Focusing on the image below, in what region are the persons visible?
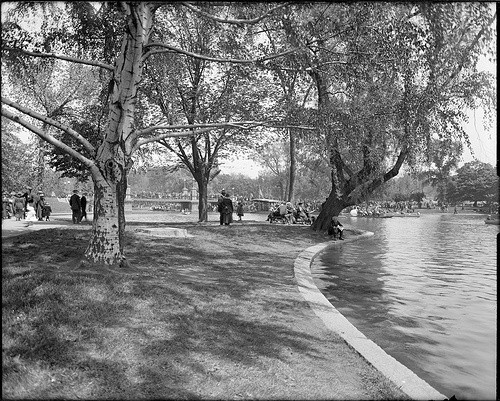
[80,192,87,222]
[354,204,415,216]
[2,187,52,221]
[328,216,345,241]
[266,201,310,224]
[236,202,244,220]
[69,189,82,224]
[217,190,233,225]
